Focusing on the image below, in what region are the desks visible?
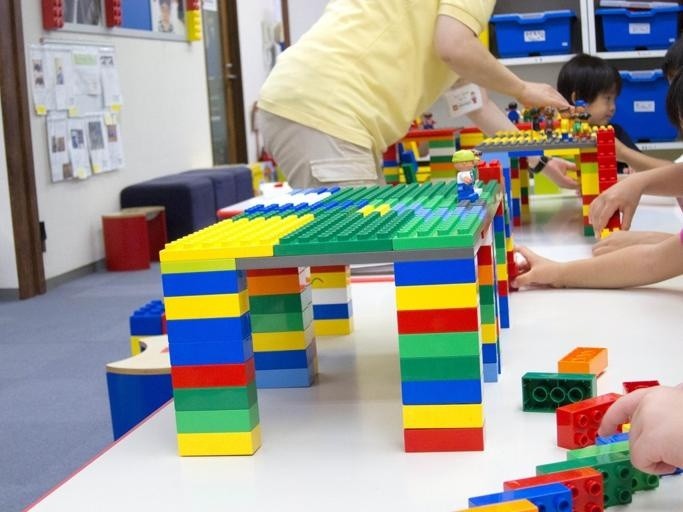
[21,177,682,511]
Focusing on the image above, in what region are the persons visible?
[512,41,683,287]
[157,0,175,33]
[616,35,683,171]
[588,67,683,257]
[557,54,641,174]
[597,382,683,474]
[256,0,581,191]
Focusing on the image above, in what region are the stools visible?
[100,166,253,272]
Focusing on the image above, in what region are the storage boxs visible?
[489,0,682,143]
